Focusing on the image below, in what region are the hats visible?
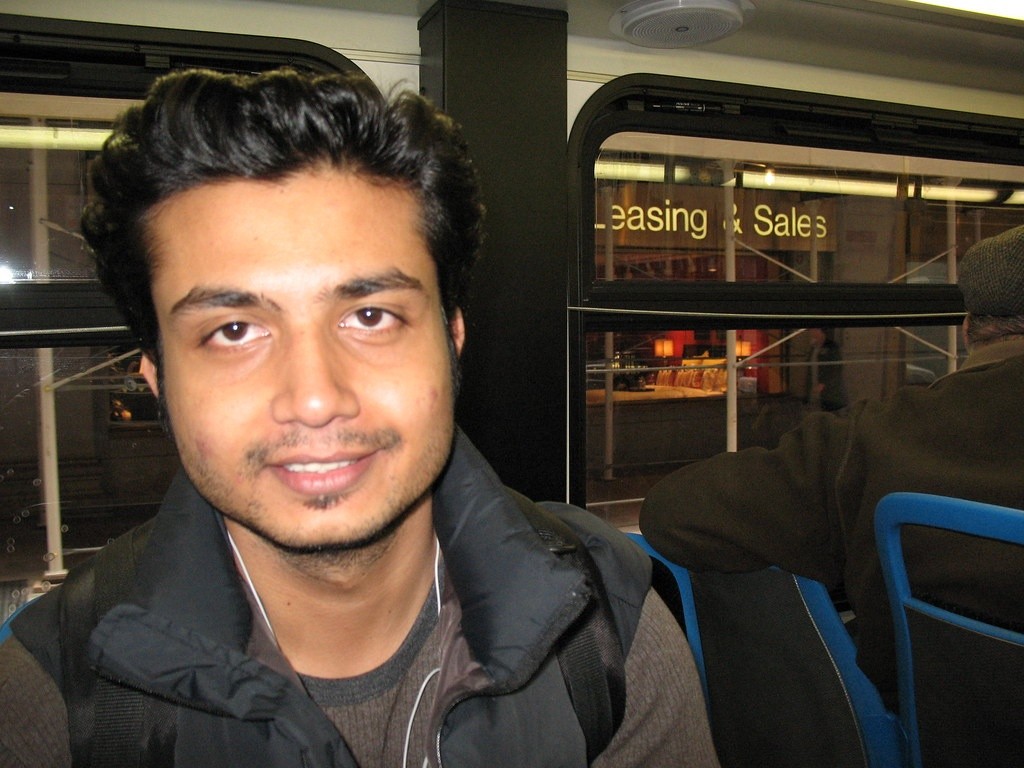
[957,225,1024,316]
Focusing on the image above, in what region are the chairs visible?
[626,491,1023,768]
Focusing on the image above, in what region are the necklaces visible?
[221,528,443,768]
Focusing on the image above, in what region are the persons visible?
[804,328,848,413]
[637,225,1024,768]
[0,71,719,768]
